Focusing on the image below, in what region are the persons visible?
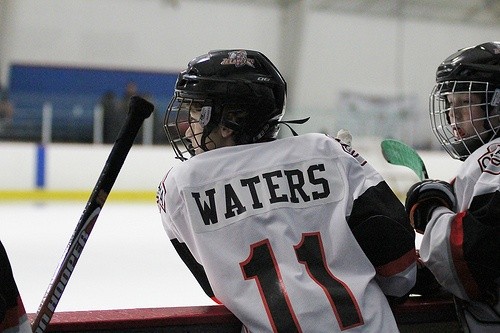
[404,41,500,333]
[102,78,144,144]
[153,47,419,333]
[0,242,34,333]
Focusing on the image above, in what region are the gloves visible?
[405,179,458,235]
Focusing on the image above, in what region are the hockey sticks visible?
[380,139,429,181]
[30,94,155,333]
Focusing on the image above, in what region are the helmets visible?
[162,49,287,162]
[429,42,500,161]
